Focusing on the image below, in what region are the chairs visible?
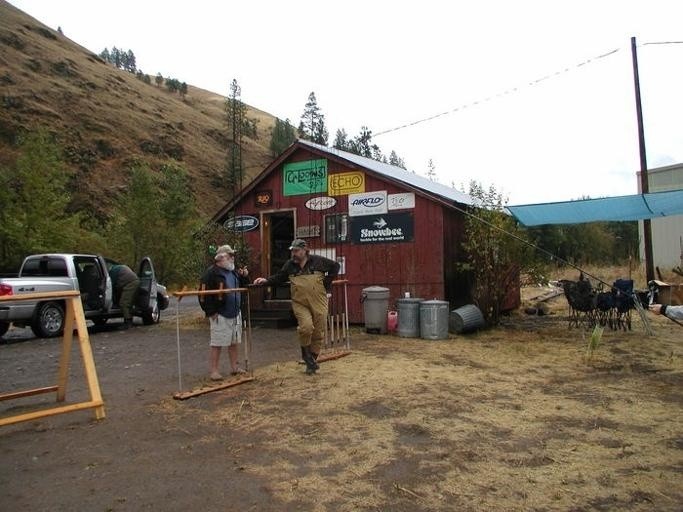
[560,278,634,332]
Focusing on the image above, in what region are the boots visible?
[301,345,318,369]
[306,352,318,375]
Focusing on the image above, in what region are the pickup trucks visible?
[0,253,169,339]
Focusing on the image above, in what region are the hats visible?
[216,245,235,254]
[289,239,305,248]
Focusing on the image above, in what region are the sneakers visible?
[210,372,221,380]
[230,368,245,373]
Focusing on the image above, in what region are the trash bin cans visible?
[450,304,483,335]
[362,285,390,334]
[396,298,450,339]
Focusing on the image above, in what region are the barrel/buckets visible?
[387,309,398,331]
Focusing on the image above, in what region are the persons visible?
[106,264,140,331]
[197,244,249,380]
[252,238,340,375]
[648,303,683,320]
